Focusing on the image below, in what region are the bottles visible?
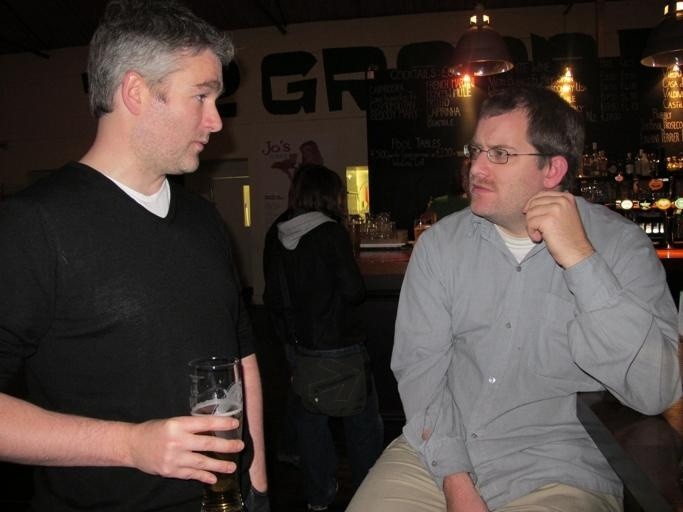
[579,141,683,243]
[351,211,395,241]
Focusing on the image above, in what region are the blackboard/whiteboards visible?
[366,53,683,242]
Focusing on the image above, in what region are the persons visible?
[659,291,683,438]
[0,6,273,510]
[262,163,385,512]
[343,82,683,512]
[428,159,469,222]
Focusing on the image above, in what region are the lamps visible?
[446,1,513,78]
[639,1,682,67]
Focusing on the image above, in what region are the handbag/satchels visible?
[288,341,370,419]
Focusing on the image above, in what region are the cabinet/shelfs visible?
[565,169,682,250]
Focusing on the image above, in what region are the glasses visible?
[463,142,553,166]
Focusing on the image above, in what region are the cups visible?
[189,355,246,511]
[414,218,433,241]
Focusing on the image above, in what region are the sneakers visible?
[308,476,339,511]
